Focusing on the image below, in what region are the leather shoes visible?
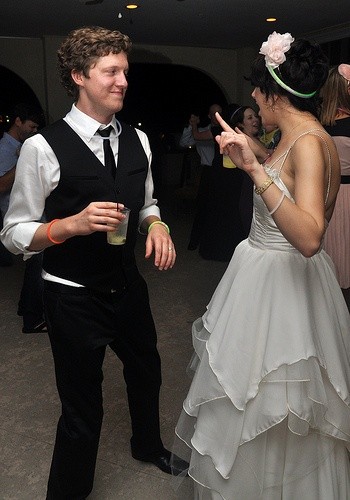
[131,441,190,477]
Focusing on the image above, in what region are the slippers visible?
[22,320,48,334]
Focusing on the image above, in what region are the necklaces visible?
[72,115,123,140]
[273,116,318,153]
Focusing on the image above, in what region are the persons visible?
[217,106,258,259]
[0,112,50,334]
[318,64,350,311]
[1,27,192,499]
[174,32,350,500]
[191,102,221,256]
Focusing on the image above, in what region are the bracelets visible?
[269,190,285,218]
[148,221,170,235]
[48,218,65,243]
[255,177,273,196]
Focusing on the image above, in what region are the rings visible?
[169,247,174,251]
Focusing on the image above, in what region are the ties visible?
[95,125,118,180]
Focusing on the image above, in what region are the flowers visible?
[259,31,294,69]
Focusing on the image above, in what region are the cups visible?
[107,207,132,246]
